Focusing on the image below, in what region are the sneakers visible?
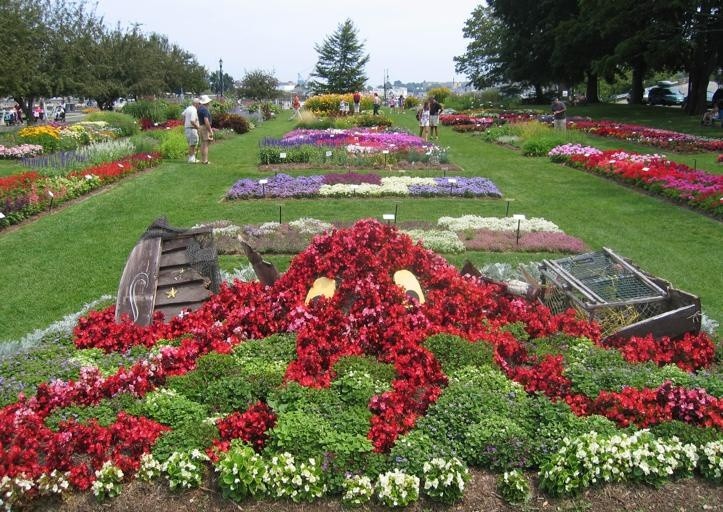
[435,136,438,139]
[202,161,211,165]
[188,160,200,163]
[430,136,433,139]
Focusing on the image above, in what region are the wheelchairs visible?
[701,105,723,128]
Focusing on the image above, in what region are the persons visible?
[399,95,406,114]
[429,98,443,139]
[372,93,380,116]
[196,94,214,164]
[551,96,567,133]
[4,101,66,126]
[288,94,302,120]
[389,96,397,114]
[703,103,719,127]
[180,98,200,163]
[339,98,348,116]
[354,90,361,115]
[418,101,432,142]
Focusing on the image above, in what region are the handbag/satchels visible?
[416,109,423,121]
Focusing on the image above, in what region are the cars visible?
[682,91,715,109]
[626,89,649,105]
[648,87,686,106]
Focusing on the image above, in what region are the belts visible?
[186,127,197,129]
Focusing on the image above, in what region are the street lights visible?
[219,59,223,96]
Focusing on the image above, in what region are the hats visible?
[199,95,212,104]
[551,96,557,101]
